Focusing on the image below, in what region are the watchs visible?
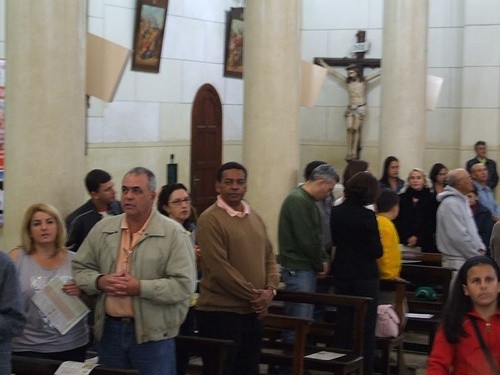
[268,287,276,296]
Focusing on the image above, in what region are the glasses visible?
[169,197,191,206]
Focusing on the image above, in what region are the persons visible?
[0,140,500,375]
[319,58,382,162]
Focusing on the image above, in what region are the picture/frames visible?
[223,6,244,79]
[132,0,169,74]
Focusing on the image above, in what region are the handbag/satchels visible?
[375,305,401,337]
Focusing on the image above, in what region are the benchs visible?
[11,264,454,375]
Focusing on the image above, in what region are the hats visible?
[414,286,435,301]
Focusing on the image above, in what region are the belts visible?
[107,315,134,323]
